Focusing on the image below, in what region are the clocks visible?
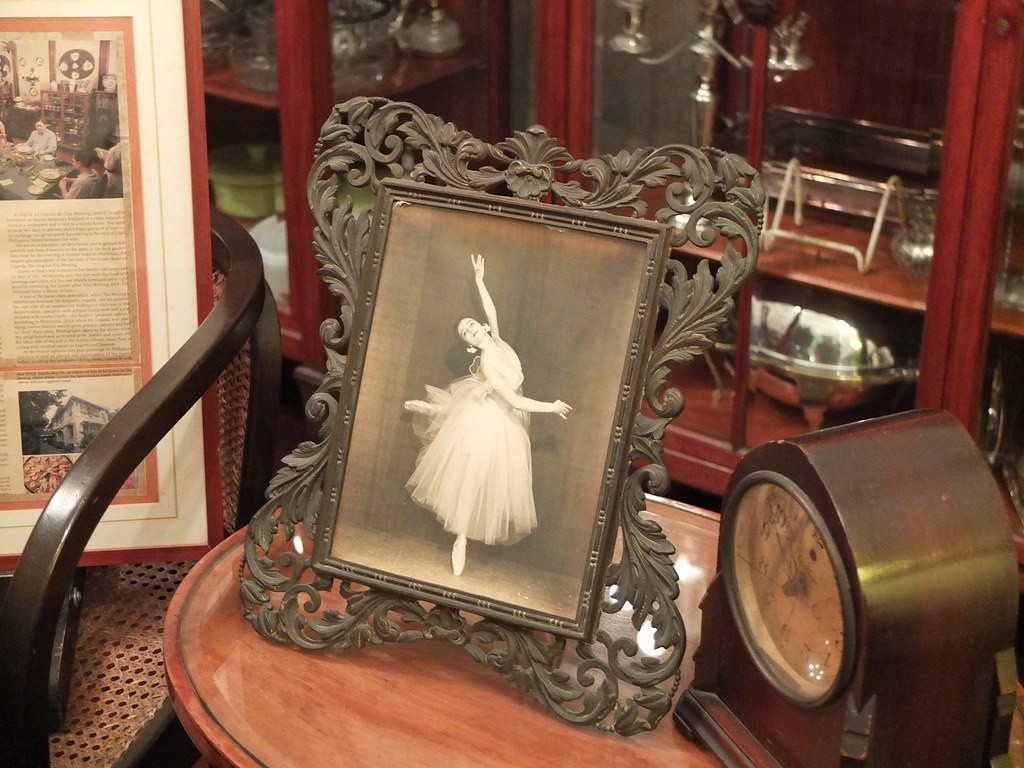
[673,407,1024,768]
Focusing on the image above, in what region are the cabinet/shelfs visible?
[203,0,513,441]
[535,0,1024,594]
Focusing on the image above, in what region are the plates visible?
[15,143,33,155]
[28,185,44,195]
[43,154,54,161]
[39,169,60,182]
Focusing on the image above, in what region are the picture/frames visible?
[237,98,765,736]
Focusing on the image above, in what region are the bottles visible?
[50,78,87,93]
[230,22,278,91]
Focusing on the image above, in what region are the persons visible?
[404,253,575,576]
[16,116,123,199]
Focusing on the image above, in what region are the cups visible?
[1,160,6,165]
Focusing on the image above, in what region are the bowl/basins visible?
[212,141,278,217]
[247,0,398,68]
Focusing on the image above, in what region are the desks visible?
[163,494,721,768]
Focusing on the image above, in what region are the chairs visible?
[0,209,283,768]
